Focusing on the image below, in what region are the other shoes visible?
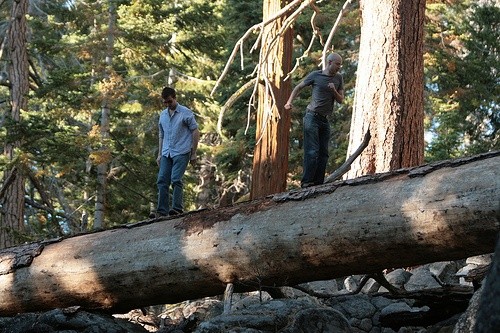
[149,211,167,219]
[169,208,183,215]
[303,182,314,187]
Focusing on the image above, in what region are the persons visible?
[284,53,345,188]
[148,87,200,218]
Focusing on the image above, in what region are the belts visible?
[309,111,329,119]
